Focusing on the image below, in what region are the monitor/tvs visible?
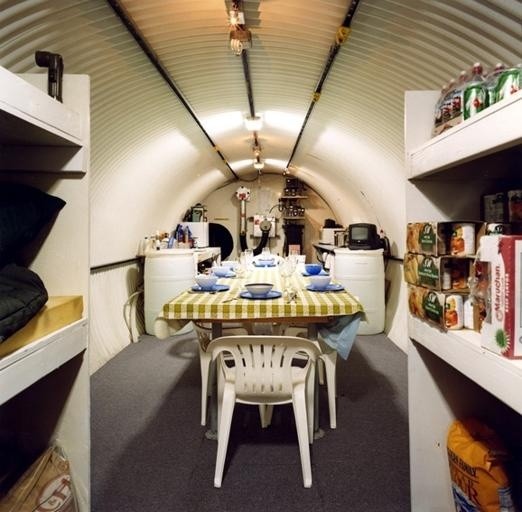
[348,222,377,250]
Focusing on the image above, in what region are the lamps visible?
[245,116,262,131]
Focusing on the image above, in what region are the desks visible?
[312,241,338,253]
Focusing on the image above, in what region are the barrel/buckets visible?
[143,250,194,336]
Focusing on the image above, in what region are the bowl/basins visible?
[245,283,274,298]
[310,276,331,290]
[195,275,218,290]
[305,265,322,275]
[212,266,230,277]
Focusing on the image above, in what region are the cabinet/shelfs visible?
[403,87,521,512]
[1,65,90,512]
[143,247,222,337]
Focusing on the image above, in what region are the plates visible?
[192,285,229,291]
[240,290,282,299]
[255,264,276,267]
[211,273,236,278]
[306,284,344,292]
[300,269,329,276]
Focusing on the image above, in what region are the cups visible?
[262,247,270,271]
[280,256,298,293]
[240,249,253,275]
[298,254,308,266]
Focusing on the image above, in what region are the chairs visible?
[193,320,214,426]
[204,334,322,490]
[317,340,339,430]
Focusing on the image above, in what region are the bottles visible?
[433,62,522,137]
[143,237,149,254]
[162,233,169,248]
[150,236,156,252]
[156,234,161,251]
